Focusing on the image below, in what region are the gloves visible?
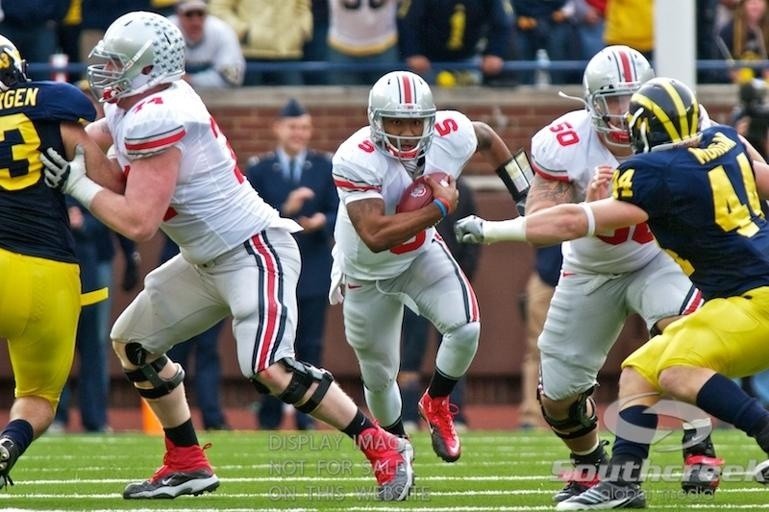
[455,215,525,244]
[39,144,104,210]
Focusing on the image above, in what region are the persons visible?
[1,1,768,512]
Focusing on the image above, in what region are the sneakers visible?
[359,388,460,501]
[681,436,721,493]
[123,438,219,498]
[555,440,645,511]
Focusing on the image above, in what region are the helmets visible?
[368,70,436,160]
[87,11,186,103]
[0,35,31,92]
[584,44,700,154]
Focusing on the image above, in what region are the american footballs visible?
[396,172,449,213]
